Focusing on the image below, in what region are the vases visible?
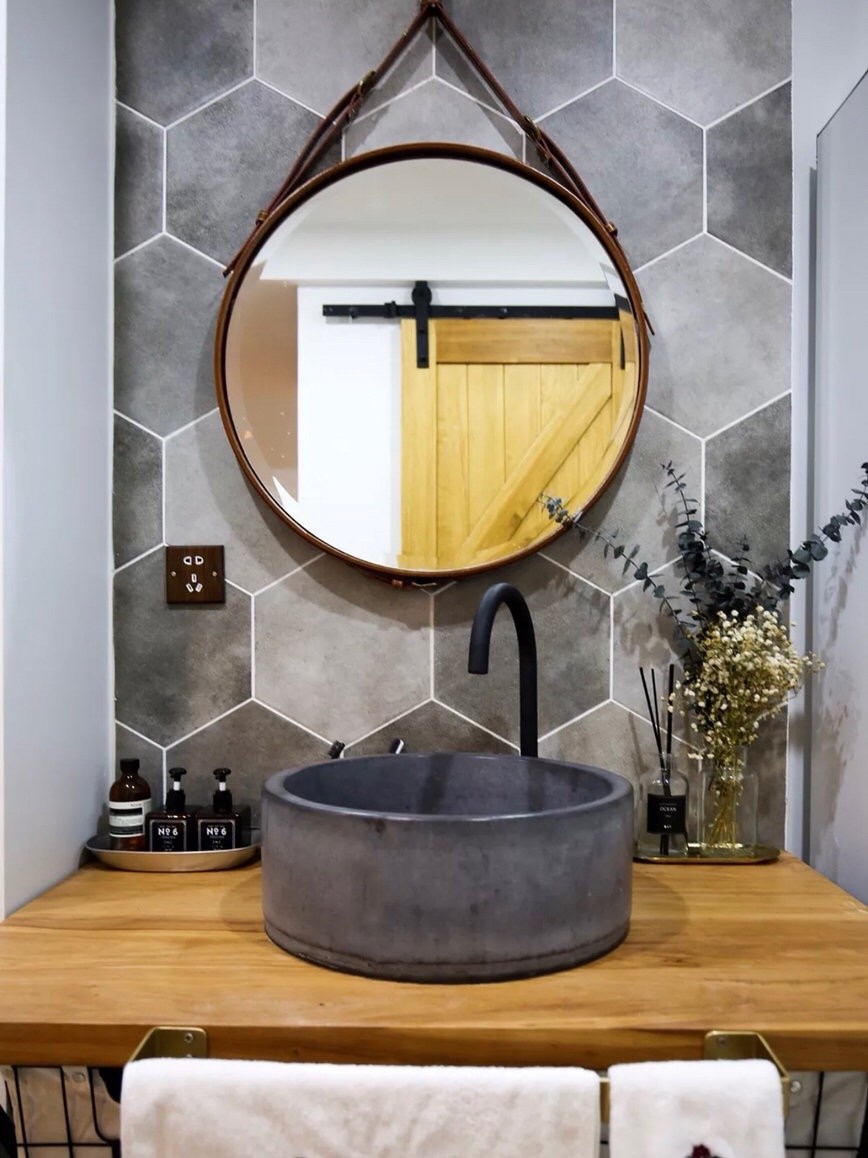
[697,746,758,857]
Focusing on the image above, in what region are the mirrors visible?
[213,0,655,590]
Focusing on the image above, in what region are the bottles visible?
[108,759,152,852]
[637,753,689,857]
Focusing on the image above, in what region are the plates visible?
[85,832,262,872]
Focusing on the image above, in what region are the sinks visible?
[255,751,642,986]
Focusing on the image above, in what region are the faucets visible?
[468,582,540,758]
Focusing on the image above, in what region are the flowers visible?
[656,604,826,849]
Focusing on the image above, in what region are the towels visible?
[118,1054,602,1158]
[602,1056,790,1158]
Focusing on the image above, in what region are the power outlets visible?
[166,545,225,604]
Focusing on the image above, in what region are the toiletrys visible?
[146,767,205,854]
[197,765,253,851]
